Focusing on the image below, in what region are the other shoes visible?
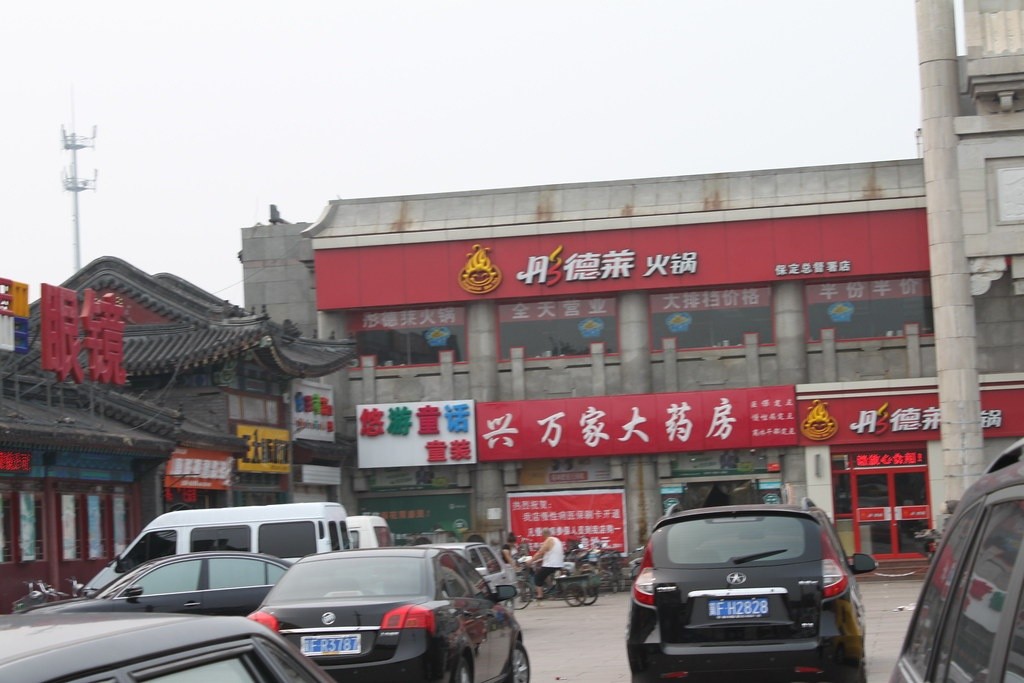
[533,597,546,600]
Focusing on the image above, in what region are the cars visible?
[16,549,293,619]
[245,546,531,683]
[0,610,336,683]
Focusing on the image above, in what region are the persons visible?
[527,528,563,599]
[499,535,517,567]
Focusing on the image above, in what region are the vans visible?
[347,515,394,549]
[83,501,353,598]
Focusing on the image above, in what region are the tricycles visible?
[508,558,597,611]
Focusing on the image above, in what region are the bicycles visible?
[12,576,84,614]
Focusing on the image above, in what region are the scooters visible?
[630,546,645,582]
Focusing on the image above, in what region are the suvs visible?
[893,438,1024,683]
[625,498,878,683]
